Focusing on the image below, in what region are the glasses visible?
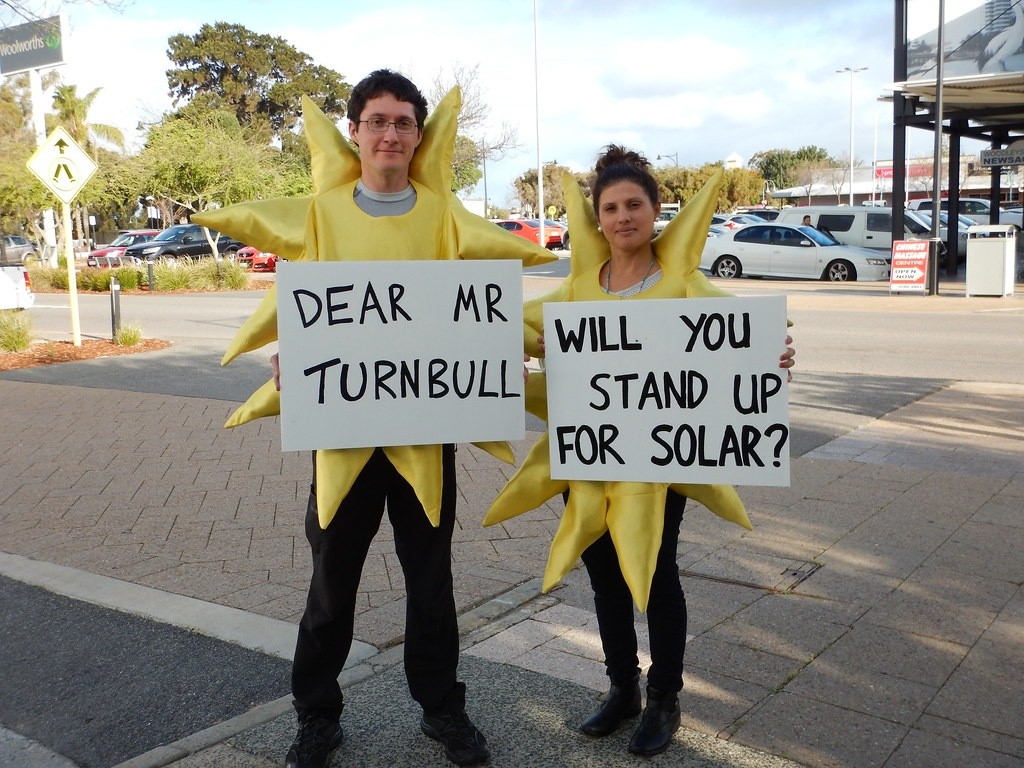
[358,117,420,134]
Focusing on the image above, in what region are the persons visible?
[536,145,796,760]
[976,0,1024,73]
[802,215,815,228]
[271,70,530,768]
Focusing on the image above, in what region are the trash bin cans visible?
[966,225,1015,298]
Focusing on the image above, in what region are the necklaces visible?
[605,257,656,295]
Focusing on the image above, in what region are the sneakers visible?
[420,709,489,767]
[284,704,344,768]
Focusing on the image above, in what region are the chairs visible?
[775,232,783,245]
[784,231,791,245]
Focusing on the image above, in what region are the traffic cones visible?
[834,65,869,206]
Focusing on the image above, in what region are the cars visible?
[651,195,1024,264]
[697,223,890,282]
[0,236,37,266]
[492,217,571,252]
[85,224,282,274]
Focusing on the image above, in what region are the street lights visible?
[656,152,680,169]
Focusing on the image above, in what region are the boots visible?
[579,670,642,736]
[629,685,681,756]
